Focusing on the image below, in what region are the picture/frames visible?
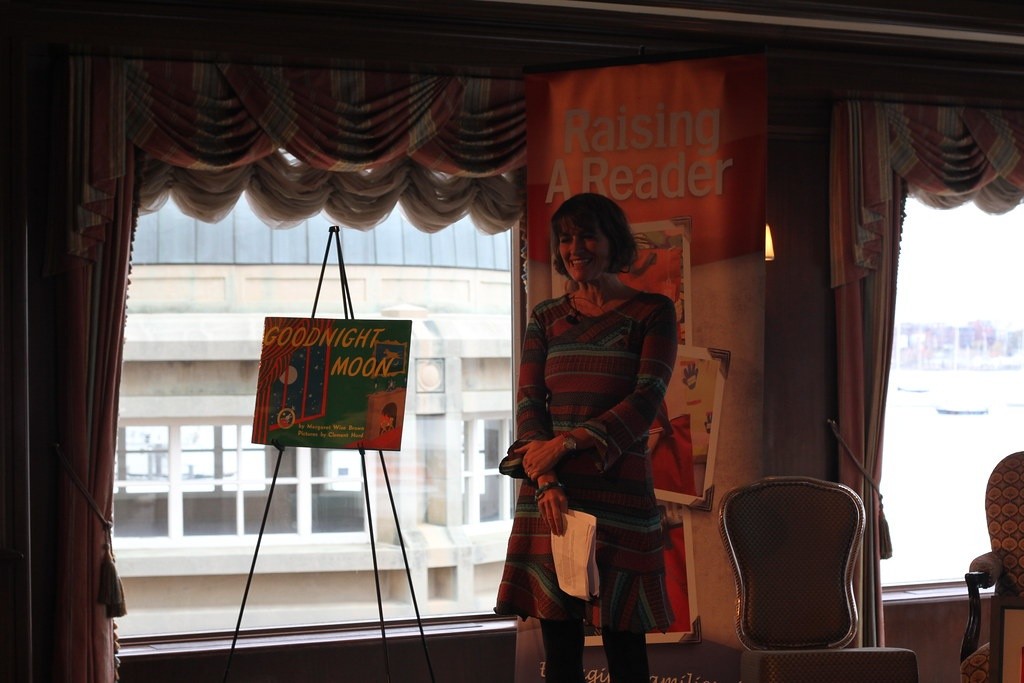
[618,216,692,347]
[584,499,701,646]
[647,342,732,512]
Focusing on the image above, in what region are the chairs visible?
[716,477,918,683]
[958,451,1024,682]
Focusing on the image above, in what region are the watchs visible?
[561,430,578,457]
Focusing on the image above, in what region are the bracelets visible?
[533,481,566,501]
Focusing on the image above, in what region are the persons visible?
[494,192,678,683]
